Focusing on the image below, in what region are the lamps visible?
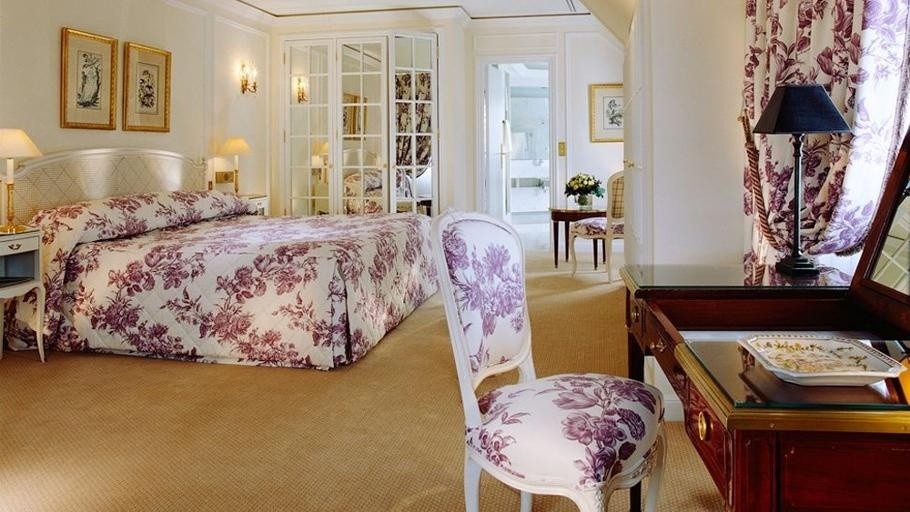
[311,152,330,184]
[751,81,852,281]
[217,135,253,196]
[239,58,258,96]
[297,76,312,104]
[1,127,46,233]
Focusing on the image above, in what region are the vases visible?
[573,191,598,211]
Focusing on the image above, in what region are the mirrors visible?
[847,124,910,335]
[281,33,437,219]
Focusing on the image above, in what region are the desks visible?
[544,204,607,272]
[611,259,908,511]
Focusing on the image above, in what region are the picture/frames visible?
[119,40,173,133]
[588,82,626,145]
[57,25,120,132]
[340,90,356,142]
[354,94,370,145]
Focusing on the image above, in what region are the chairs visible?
[427,205,672,512]
[566,168,624,285]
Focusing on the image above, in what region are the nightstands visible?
[0,219,47,368]
[235,190,270,216]
[311,180,332,215]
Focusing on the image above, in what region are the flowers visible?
[563,170,608,204]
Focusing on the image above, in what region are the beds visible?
[310,146,429,217]
[1,145,441,372]
